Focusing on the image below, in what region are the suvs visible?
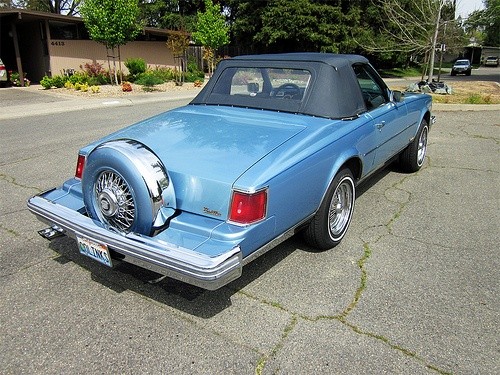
[451,59,471,76]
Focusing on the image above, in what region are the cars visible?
[27,52,435,290]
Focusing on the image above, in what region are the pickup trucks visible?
[486,56,499,66]
